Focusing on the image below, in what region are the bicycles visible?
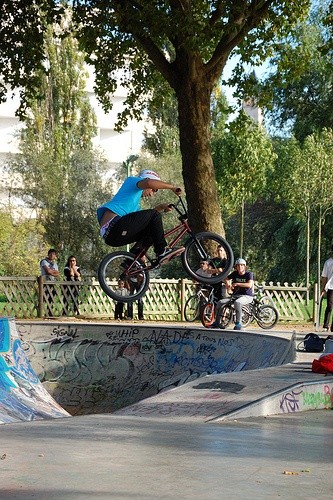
[97,188,235,302]
[184,283,279,329]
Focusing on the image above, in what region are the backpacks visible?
[298,333,324,353]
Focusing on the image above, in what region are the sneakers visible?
[208,321,220,328]
[322,327,327,333]
[218,322,225,329]
[156,246,186,266]
[234,322,242,330]
[119,259,148,274]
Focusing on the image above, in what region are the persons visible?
[37,248,60,317]
[320,245,333,332]
[63,256,81,316]
[96,170,185,271]
[113,245,255,330]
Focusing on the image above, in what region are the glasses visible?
[199,263,207,265]
[69,260,77,262]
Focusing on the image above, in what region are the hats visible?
[137,169,161,182]
[234,258,247,268]
